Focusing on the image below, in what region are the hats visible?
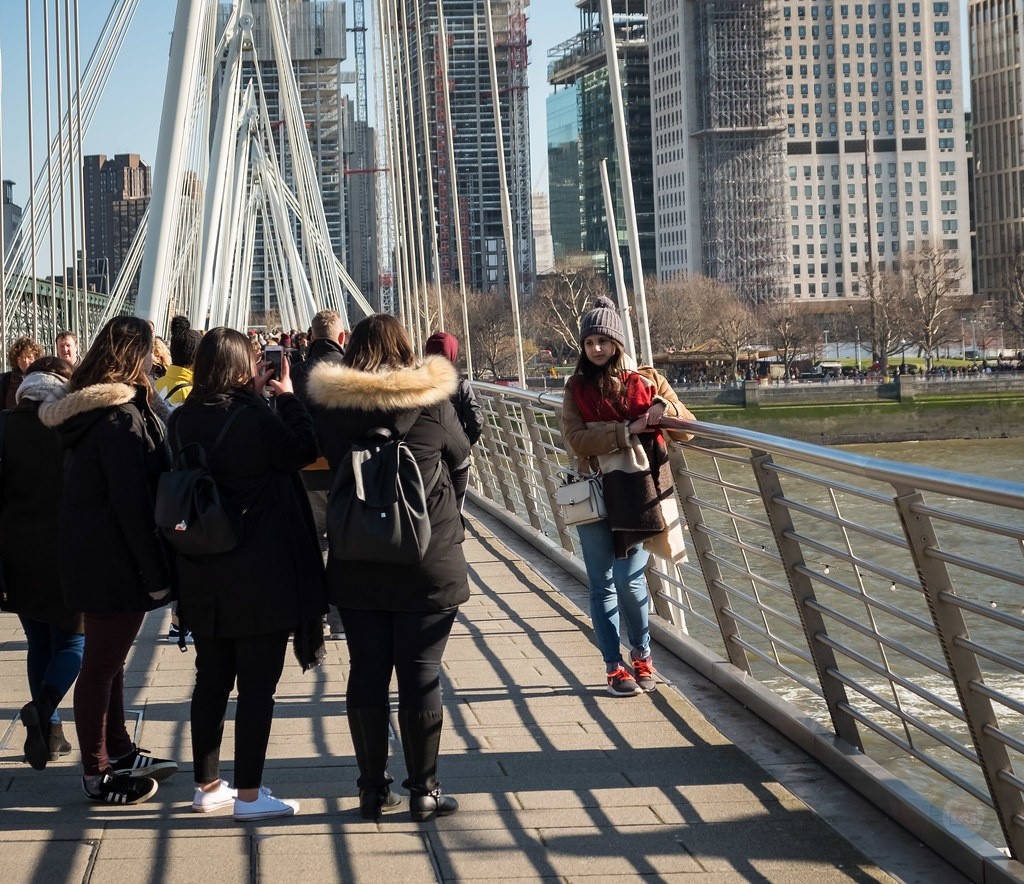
[579,296,625,348]
[247,331,255,335]
[170,316,203,369]
[280,333,290,347]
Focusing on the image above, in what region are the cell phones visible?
[264,346,284,391]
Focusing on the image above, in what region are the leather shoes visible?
[401,779,459,822]
[357,774,402,819]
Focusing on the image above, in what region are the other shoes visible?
[330,631,347,640]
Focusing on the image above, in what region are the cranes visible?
[352,0,371,302]
[509,0,535,301]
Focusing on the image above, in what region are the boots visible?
[19,684,64,770]
[47,722,73,761]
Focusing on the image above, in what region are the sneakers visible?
[168,623,195,642]
[607,663,643,696]
[79,766,159,806]
[630,649,657,692]
[232,786,300,821]
[192,779,238,813]
[107,743,178,783]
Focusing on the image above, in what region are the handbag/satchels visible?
[556,468,608,526]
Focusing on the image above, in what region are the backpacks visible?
[154,401,248,555]
[326,406,443,565]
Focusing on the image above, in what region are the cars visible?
[965,350,980,360]
[539,349,553,362]
[828,366,863,377]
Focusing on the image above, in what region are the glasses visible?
[297,337,305,341]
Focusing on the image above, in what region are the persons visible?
[679,358,991,387]
[0,311,486,823]
[561,296,696,696]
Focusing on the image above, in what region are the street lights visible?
[961,318,966,360]
[900,338,908,374]
[970,320,976,363]
[999,322,1004,362]
[855,325,861,371]
[824,330,829,359]
